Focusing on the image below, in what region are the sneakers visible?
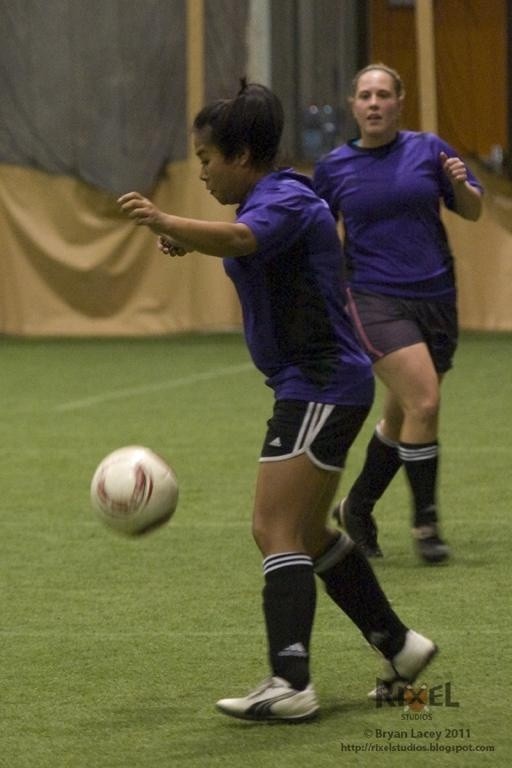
[331,496,386,559]
[410,522,452,565]
[211,674,324,724]
[366,626,440,703]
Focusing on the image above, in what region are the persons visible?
[111,75,442,728]
[313,63,487,568]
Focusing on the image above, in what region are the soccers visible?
[89,446,179,537]
